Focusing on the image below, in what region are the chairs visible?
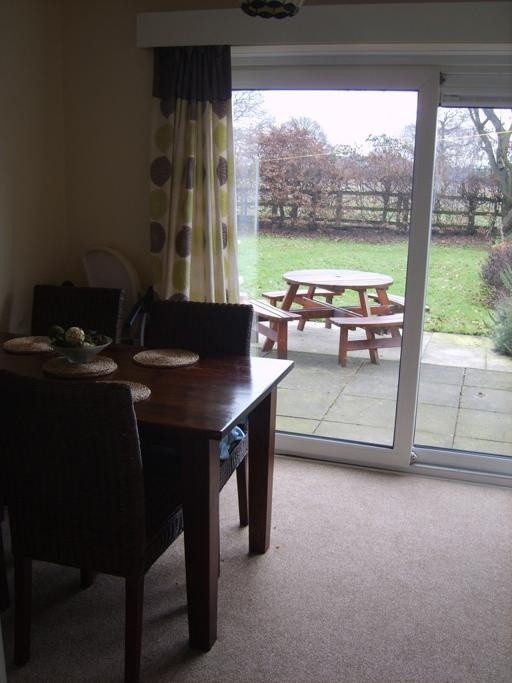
[31,281,124,343]
[12,370,196,680]
[140,298,258,579]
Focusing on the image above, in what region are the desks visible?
[2,332,296,653]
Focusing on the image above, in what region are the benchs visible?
[242,268,431,365]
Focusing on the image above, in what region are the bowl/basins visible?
[48,336,113,363]
